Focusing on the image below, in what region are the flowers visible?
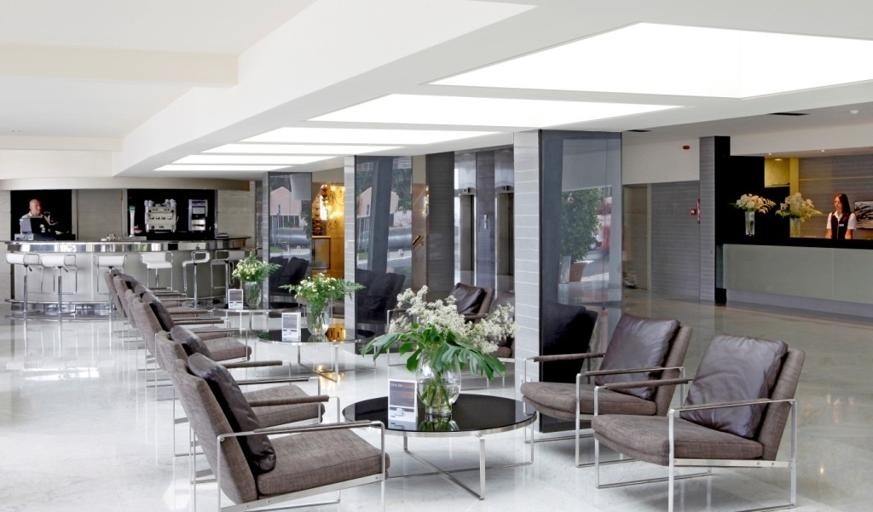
[230,252,281,281]
[733,190,774,215]
[363,287,515,375]
[775,192,821,221]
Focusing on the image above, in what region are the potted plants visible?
[558,186,611,283]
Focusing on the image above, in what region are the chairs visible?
[386,282,494,369]
[588,333,804,511]
[517,311,691,468]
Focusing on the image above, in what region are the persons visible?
[824,192,858,239]
[20,198,48,223]
[393,203,412,256]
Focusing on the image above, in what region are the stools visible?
[4,252,42,324]
[211,247,242,299]
[138,249,174,291]
[37,250,82,320]
[91,253,124,317]
[180,246,209,299]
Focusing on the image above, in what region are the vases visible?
[745,209,756,238]
[789,216,800,237]
[416,352,464,415]
